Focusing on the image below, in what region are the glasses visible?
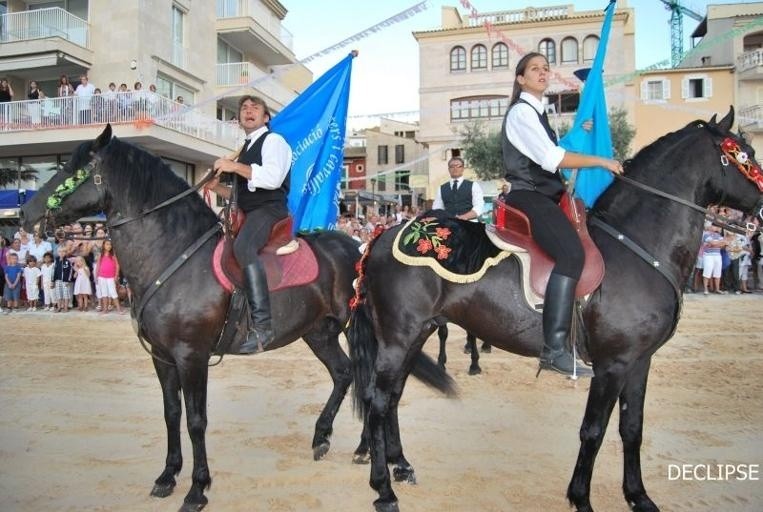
[450,164,463,168]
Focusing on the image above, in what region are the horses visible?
[345,103,763,512]
[18,121,456,512]
[437,321,492,376]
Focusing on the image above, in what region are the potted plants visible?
[449,105,505,199]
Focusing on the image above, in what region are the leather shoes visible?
[743,291,752,293]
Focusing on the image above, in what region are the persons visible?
[691,202,762,294]
[28,77,194,125]
[478,211,492,224]
[0,79,14,129]
[497,184,510,201]
[430,157,484,225]
[501,52,628,380]
[203,96,291,355]
[336,197,421,245]
[0,218,132,313]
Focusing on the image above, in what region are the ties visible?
[239,139,251,160]
[453,180,459,192]
[542,109,549,126]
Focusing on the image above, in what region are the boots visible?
[538,272,595,377]
[239,262,273,354]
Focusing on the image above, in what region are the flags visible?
[267,55,356,236]
[560,2,621,218]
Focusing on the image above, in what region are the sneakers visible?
[704,290,708,295]
[717,290,724,295]
